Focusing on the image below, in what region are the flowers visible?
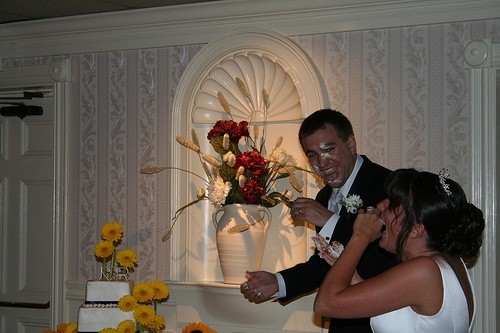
[98,278,169,333]
[44,321,78,333]
[182,321,218,333]
[94,221,138,282]
[140,77,318,242]
[338,193,364,214]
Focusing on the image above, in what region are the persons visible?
[240,108,402,333]
[313,167,485,333]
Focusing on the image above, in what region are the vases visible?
[212,203,272,284]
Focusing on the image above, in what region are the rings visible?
[366,206,374,212]
[298,207,301,214]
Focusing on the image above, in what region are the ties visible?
[329,191,342,214]
